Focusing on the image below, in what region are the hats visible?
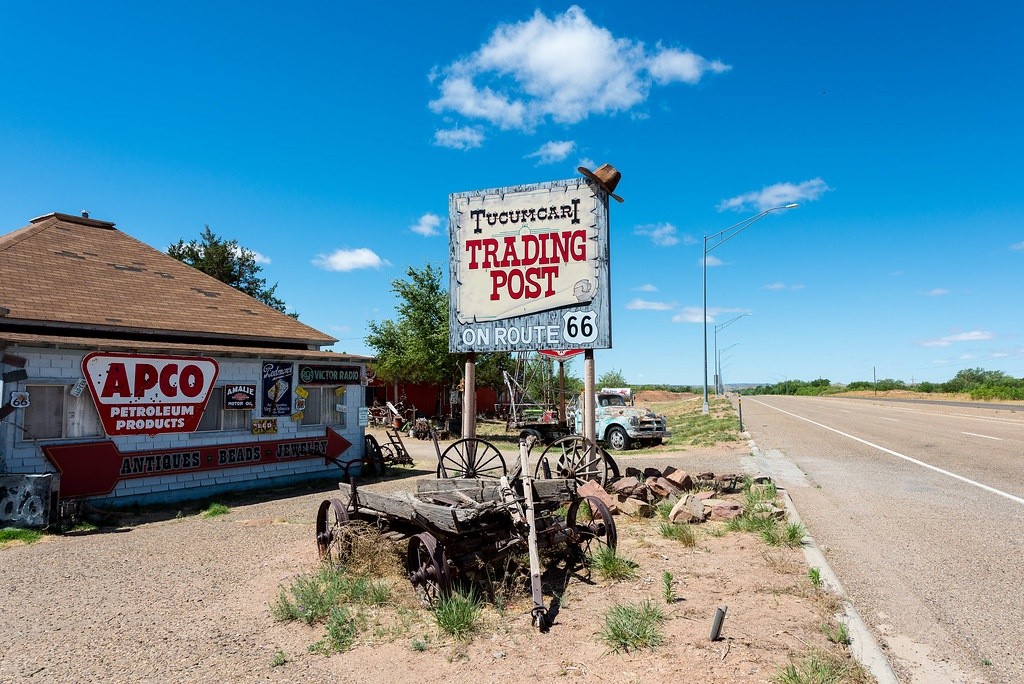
[578,164,624,203]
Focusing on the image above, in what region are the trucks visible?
[514,391,666,451]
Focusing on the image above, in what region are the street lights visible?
[701,202,799,415]
[714,312,753,396]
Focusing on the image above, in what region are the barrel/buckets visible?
[410,429,416,438]
